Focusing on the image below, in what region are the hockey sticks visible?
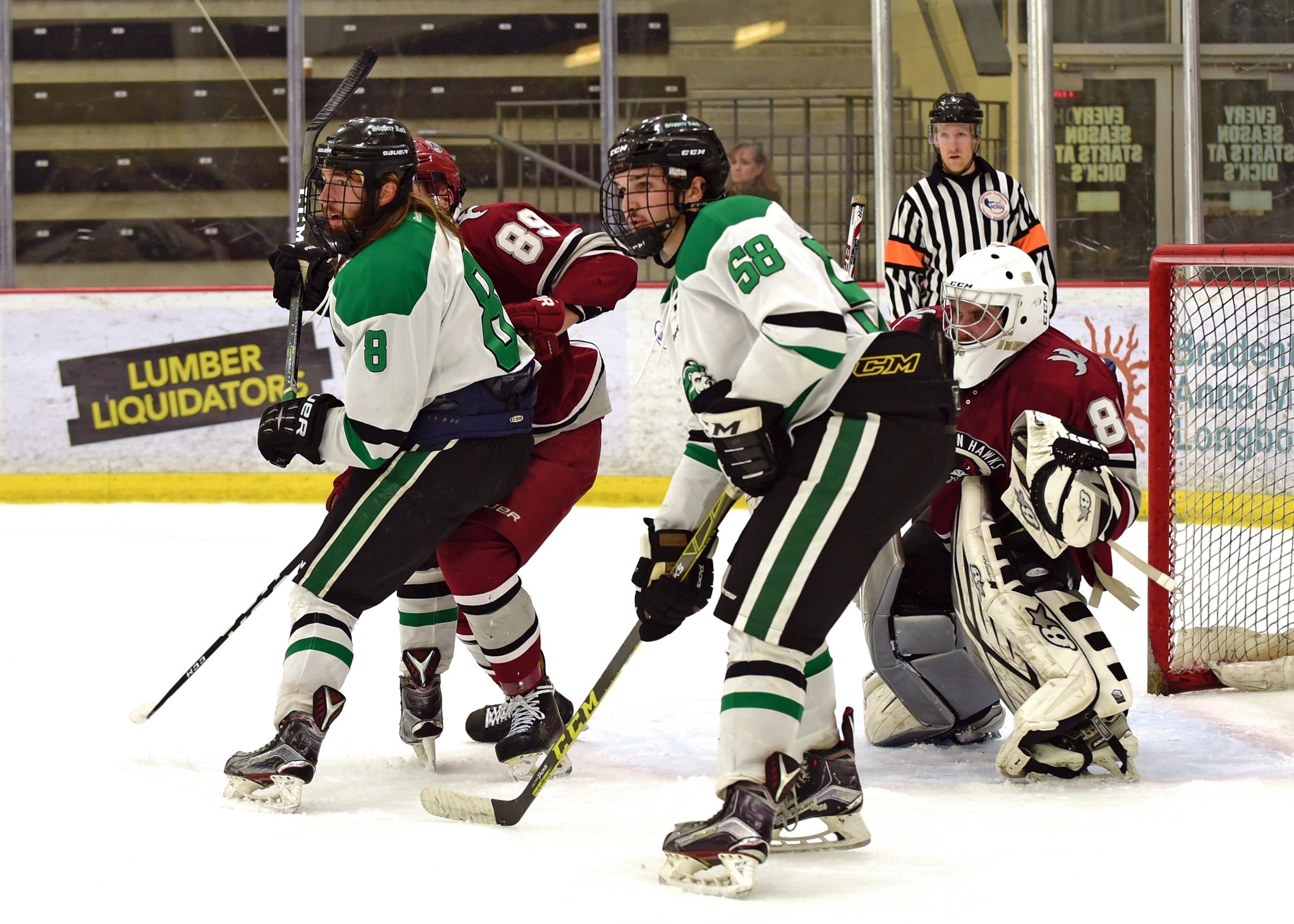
[844,194,867,280]
[147,543,309,717]
[282,45,380,402]
[420,481,742,827]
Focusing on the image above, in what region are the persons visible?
[225,116,543,788]
[326,136,638,764]
[598,113,954,865]
[884,92,1058,323]
[852,243,1143,784]
[724,137,782,207]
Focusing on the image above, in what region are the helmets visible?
[599,112,731,259]
[941,243,1050,389]
[930,93,984,125]
[302,117,418,253]
[413,137,467,218]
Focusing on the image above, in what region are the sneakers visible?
[768,706,871,853]
[399,646,443,771]
[224,686,346,814]
[659,751,801,898]
[465,687,573,742]
[495,649,572,781]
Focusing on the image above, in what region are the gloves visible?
[268,243,339,316]
[633,517,714,641]
[693,380,790,497]
[506,295,563,360]
[258,394,344,468]
